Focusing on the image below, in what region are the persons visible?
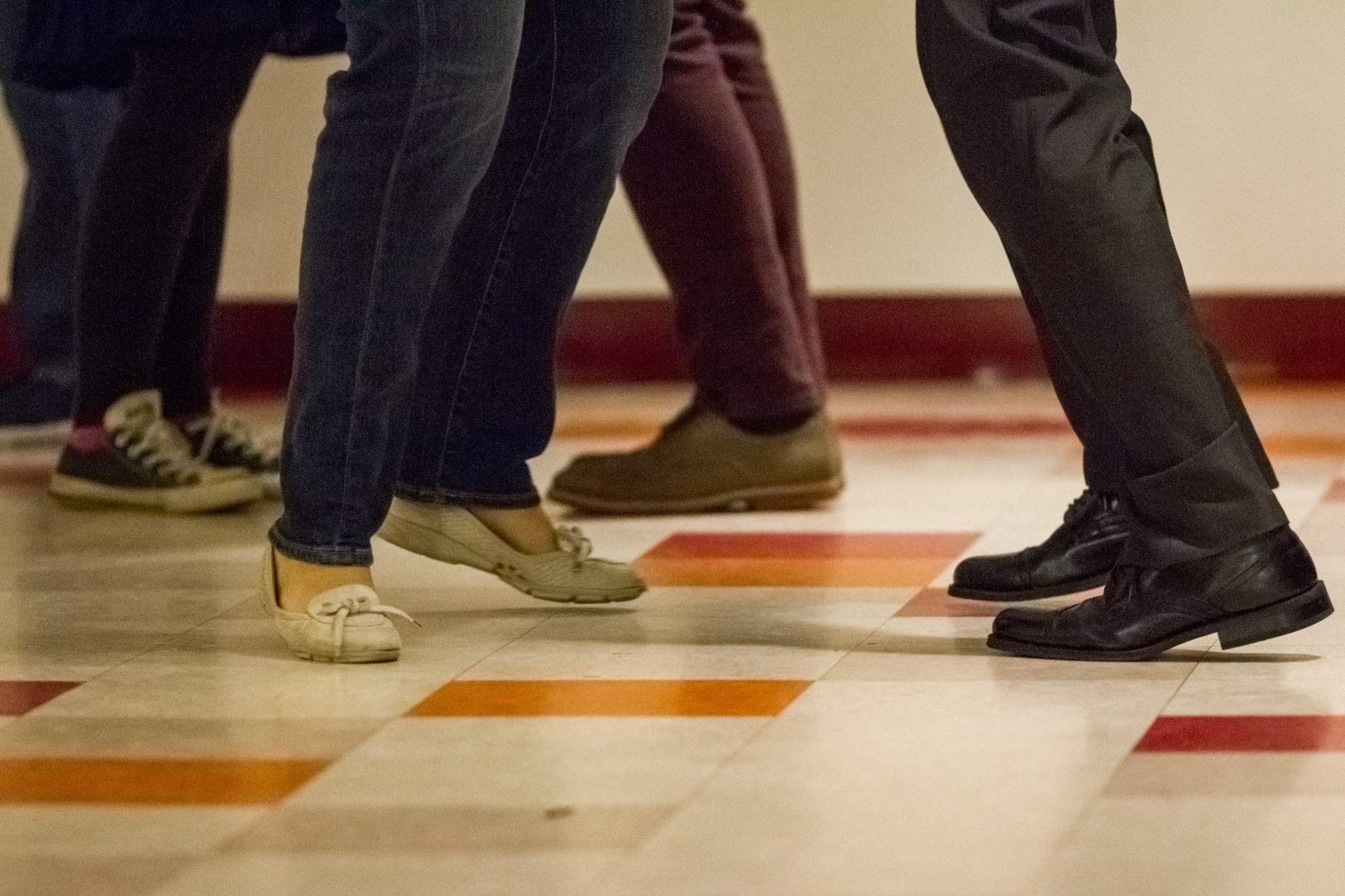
[0,0,291,517]
[913,0,1336,665]
[536,0,847,513]
[261,0,675,664]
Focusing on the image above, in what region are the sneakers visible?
[547,403,843,513]
[49,390,265,512]
[186,394,280,490]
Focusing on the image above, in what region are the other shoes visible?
[260,542,422,662]
[377,497,644,604]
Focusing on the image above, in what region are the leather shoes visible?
[948,484,1140,601]
[987,523,1335,663]
